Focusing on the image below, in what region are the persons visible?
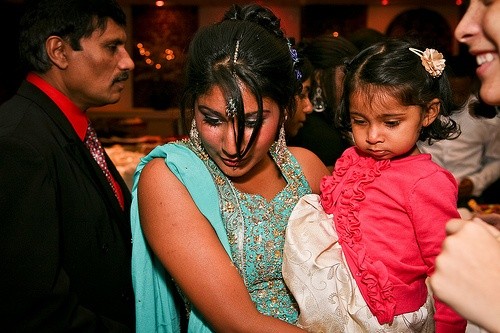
[0,0,136,333]
[129,2,331,332]
[384,0,500,208]
[285,29,353,170]
[428,0,500,332]
[282,40,468,332]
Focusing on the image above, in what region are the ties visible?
[87,124,124,210]
